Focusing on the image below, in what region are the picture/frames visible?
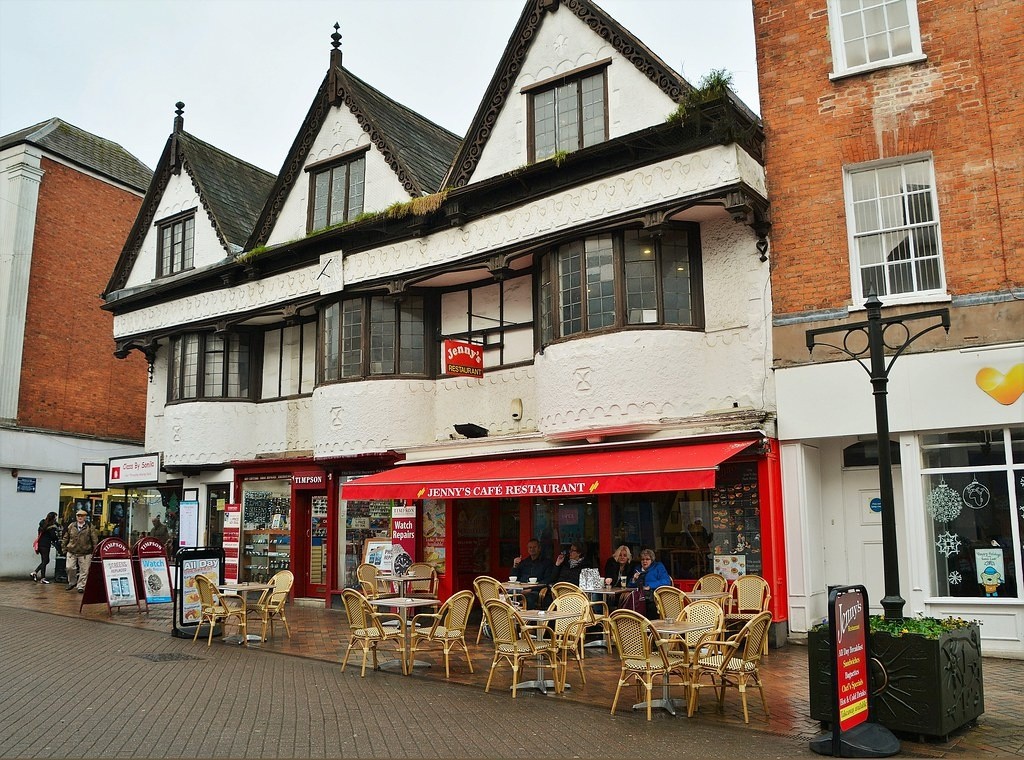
[360,538,393,574]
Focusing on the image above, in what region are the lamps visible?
[453,423,488,439]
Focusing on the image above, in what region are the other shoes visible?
[595,627,603,639]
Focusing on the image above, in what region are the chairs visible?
[473,576,613,698]
[608,574,772,724]
[341,563,475,678]
[247,570,295,641]
[193,574,247,647]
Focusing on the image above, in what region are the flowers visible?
[809,611,984,640]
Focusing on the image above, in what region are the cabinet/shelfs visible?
[243,530,291,583]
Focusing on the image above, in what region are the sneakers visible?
[40,578,49,584]
[31,572,38,582]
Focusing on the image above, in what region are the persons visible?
[592,545,632,613]
[61,510,97,592]
[628,549,671,620]
[689,517,713,574]
[150,517,169,545]
[544,541,587,608]
[510,539,553,609]
[30,512,63,585]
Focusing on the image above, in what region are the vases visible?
[809,622,983,742]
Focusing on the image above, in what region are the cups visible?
[408,571,415,575]
[509,577,517,581]
[529,577,537,583]
[621,577,626,588]
[599,578,605,587]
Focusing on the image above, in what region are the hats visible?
[695,517,703,522]
[76,509,87,516]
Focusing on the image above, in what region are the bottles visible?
[621,528,625,540]
[270,560,289,569]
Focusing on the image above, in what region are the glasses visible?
[641,558,651,561]
[569,549,577,552]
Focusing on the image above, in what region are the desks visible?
[216,582,276,644]
[681,592,730,654]
[633,620,715,715]
[510,610,581,694]
[582,587,638,650]
[368,598,441,671]
[499,582,546,639]
[375,576,432,626]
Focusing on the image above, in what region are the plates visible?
[185,579,198,589]
[528,582,539,584]
[509,581,521,583]
[185,594,201,604]
[186,610,201,620]
[407,575,417,577]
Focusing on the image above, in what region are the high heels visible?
[586,628,592,637]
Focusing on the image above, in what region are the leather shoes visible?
[78,586,85,593]
[65,583,77,590]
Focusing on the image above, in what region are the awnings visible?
[338,437,761,502]
